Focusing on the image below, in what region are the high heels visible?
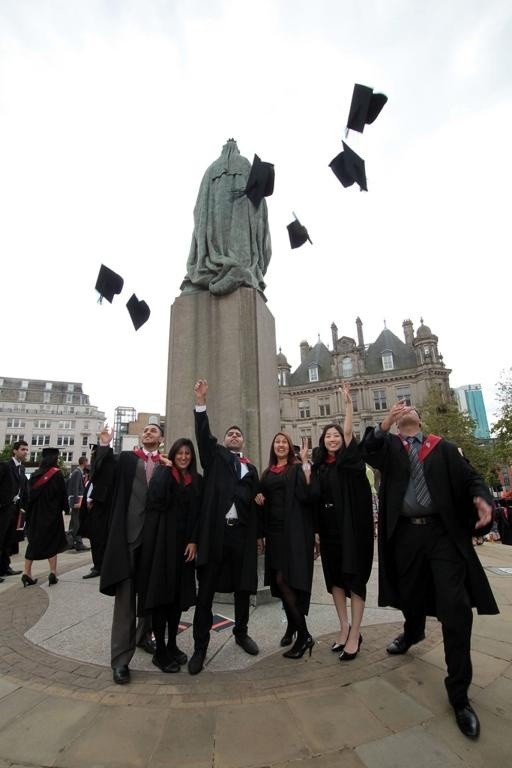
[283,635,315,659]
[48,573,58,586]
[21,575,37,588]
[339,632,363,661]
[331,624,351,651]
[281,626,296,647]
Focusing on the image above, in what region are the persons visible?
[82,469,89,487]
[180,138,271,296]
[76,481,101,579]
[0,441,29,584]
[188,379,260,676]
[147,437,203,673]
[252,433,314,659]
[88,422,167,686]
[358,398,499,741]
[21,447,70,589]
[310,380,374,661]
[67,457,91,552]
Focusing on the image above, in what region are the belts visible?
[225,517,238,526]
[404,516,431,526]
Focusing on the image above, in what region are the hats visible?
[94,263,124,305]
[38,445,65,456]
[244,153,275,210]
[287,211,313,250]
[328,140,368,193]
[346,84,388,135]
[126,293,151,331]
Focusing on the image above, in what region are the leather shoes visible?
[82,570,99,579]
[113,664,130,683]
[6,567,23,575]
[142,639,156,655]
[386,631,426,655]
[454,701,481,742]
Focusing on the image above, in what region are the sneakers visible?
[75,543,91,551]
[152,650,180,673]
[188,653,206,675]
[235,635,259,656]
[166,643,187,665]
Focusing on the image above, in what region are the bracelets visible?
[302,462,309,470]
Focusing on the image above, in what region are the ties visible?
[406,438,431,508]
[145,453,154,487]
[234,454,241,480]
